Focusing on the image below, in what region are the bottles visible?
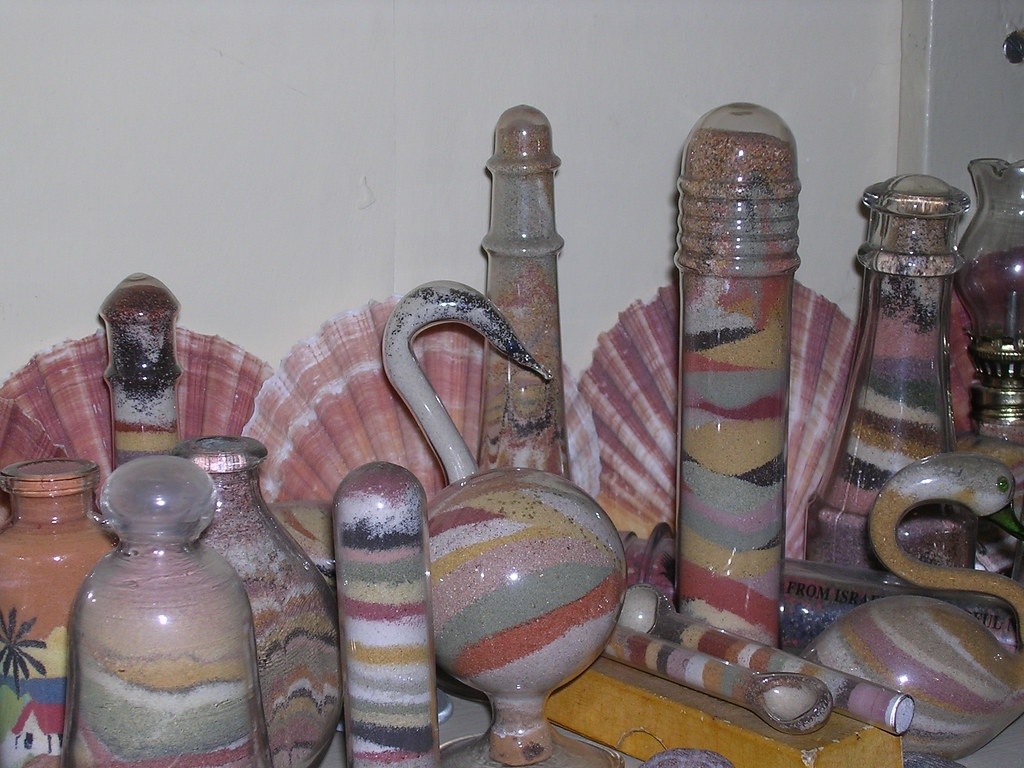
[806,175,978,599]
[71,459,270,768]
[0,459,121,768]
[175,435,341,768]
